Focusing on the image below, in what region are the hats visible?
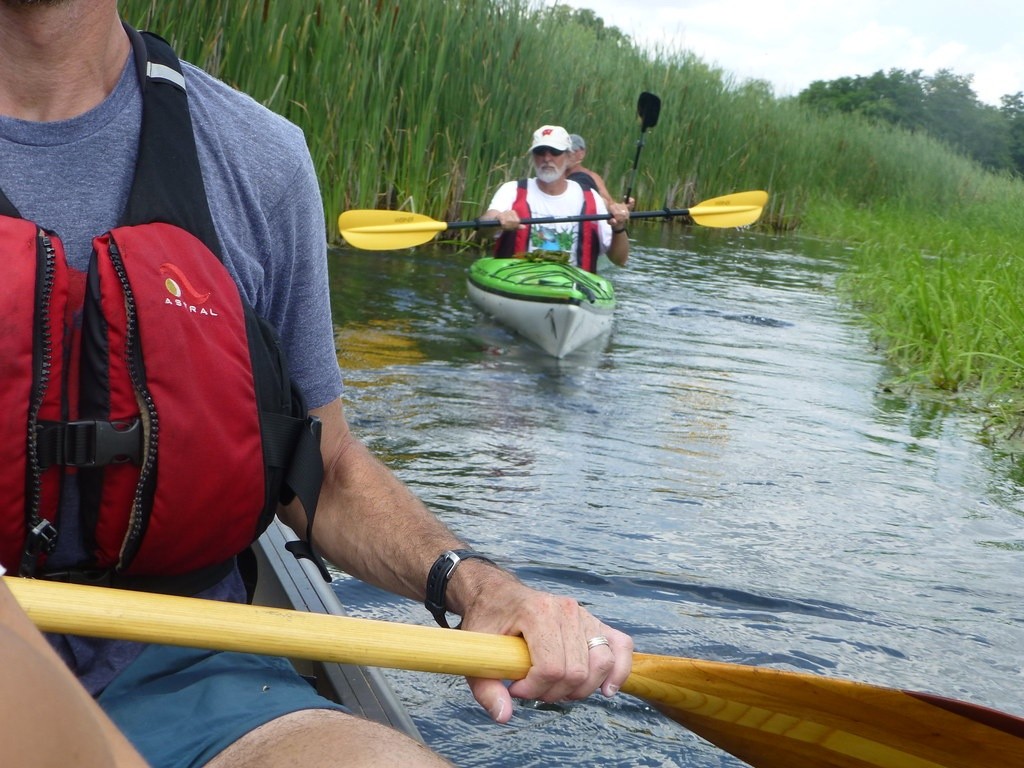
[529,125,574,154]
[568,133,586,151]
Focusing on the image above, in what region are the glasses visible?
[533,146,568,157]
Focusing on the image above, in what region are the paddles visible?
[1,573,1024,768]
[339,187,771,251]
[623,89,663,205]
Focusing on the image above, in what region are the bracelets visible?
[614,227,626,234]
[425,547,499,630]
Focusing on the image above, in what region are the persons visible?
[475,124,631,275]
[0,0,632,768]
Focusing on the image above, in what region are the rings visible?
[622,210,625,213]
[587,636,608,651]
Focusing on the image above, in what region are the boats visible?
[465,249,617,358]
